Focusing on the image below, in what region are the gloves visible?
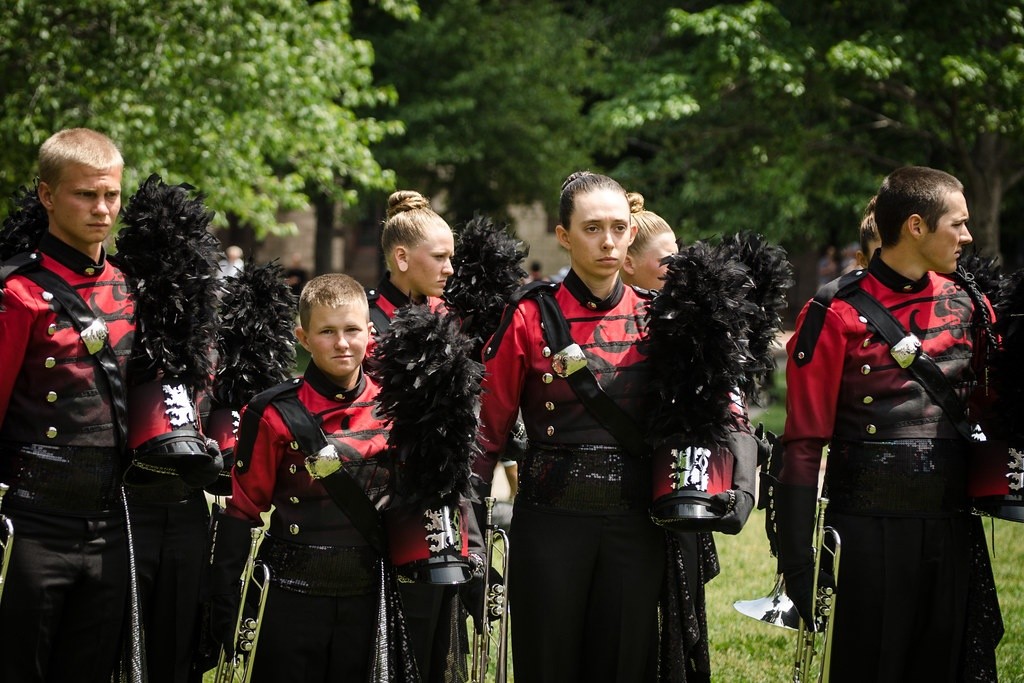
[208,512,261,660]
[761,481,839,634]
[458,479,504,635]
[708,429,758,535]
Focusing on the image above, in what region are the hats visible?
[124,367,220,491]
[387,501,473,585]
[648,443,735,534]
[205,404,243,477]
[964,449,1024,523]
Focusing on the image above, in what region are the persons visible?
[0,128,222,683]
[209,165,1023,683]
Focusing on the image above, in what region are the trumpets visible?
[471,494,510,683]
[730,493,841,683]
[216,525,270,683]
[0,478,15,607]
